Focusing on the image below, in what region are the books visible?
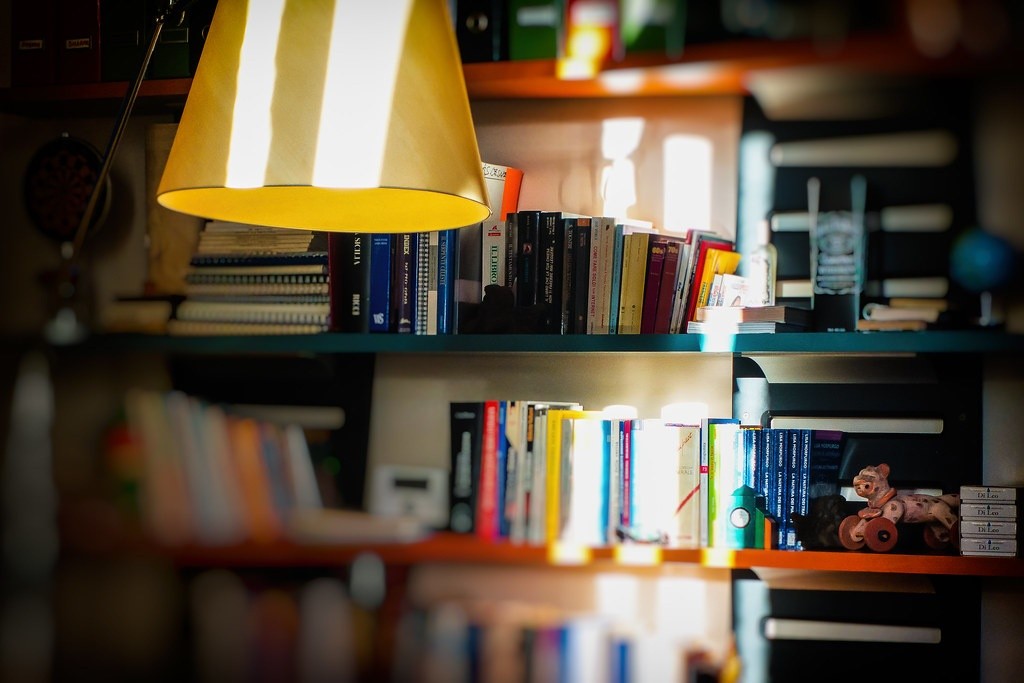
[122,156,949,683]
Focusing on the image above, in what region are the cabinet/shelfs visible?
[0,0,1024,683]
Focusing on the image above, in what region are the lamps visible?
[154,0,493,234]
